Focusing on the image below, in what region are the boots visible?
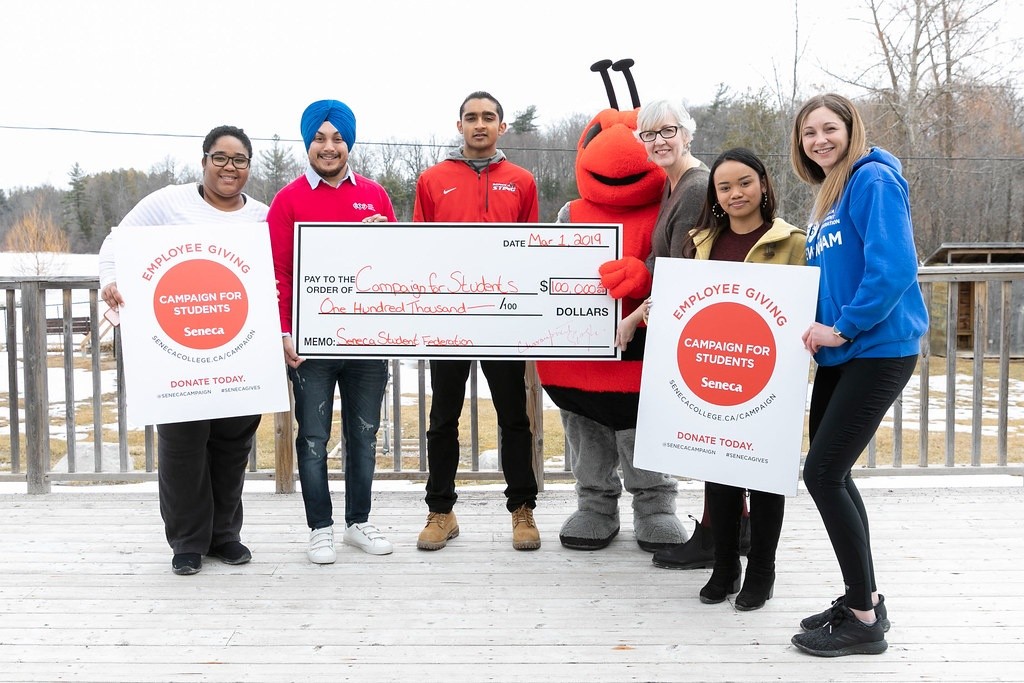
[735,492,786,610]
[699,478,742,604]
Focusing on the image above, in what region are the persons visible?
[266,100,398,565]
[791,94,930,657]
[631,98,752,572]
[98,126,270,574]
[644,147,808,609]
[411,92,540,550]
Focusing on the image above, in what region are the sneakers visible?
[510,505,541,551]
[792,604,887,655]
[801,597,891,632]
[309,526,337,564]
[342,521,393,555]
[209,542,251,565]
[417,508,459,550]
[172,546,202,575]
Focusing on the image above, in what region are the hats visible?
[300,99,355,153]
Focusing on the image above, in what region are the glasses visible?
[639,126,683,142]
[205,151,251,170]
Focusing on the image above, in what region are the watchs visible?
[833,325,852,342]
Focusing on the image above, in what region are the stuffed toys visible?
[537,59,691,552]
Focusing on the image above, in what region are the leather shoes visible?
[653,514,715,570]
[739,512,752,558]
